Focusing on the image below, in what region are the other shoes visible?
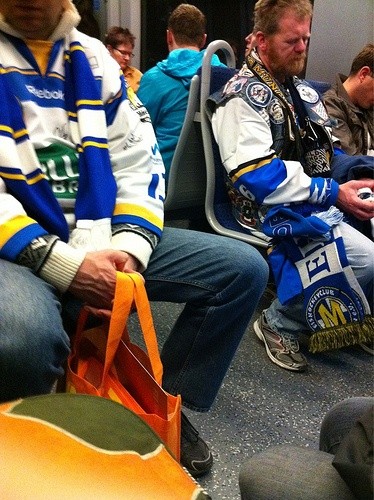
[181,409,214,475]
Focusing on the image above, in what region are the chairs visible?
[158,68,208,220]
[202,38,280,303]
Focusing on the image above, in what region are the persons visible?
[221,37,238,68]
[320,42,374,157]
[102,26,144,96]
[0,0,271,477]
[243,32,259,58]
[205,0,373,371]
[239,397,374,500]
[137,4,227,198]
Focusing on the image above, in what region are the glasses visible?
[114,47,135,58]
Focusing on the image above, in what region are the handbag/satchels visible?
[66,270,181,463]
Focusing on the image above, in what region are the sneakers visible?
[253,312,309,370]
